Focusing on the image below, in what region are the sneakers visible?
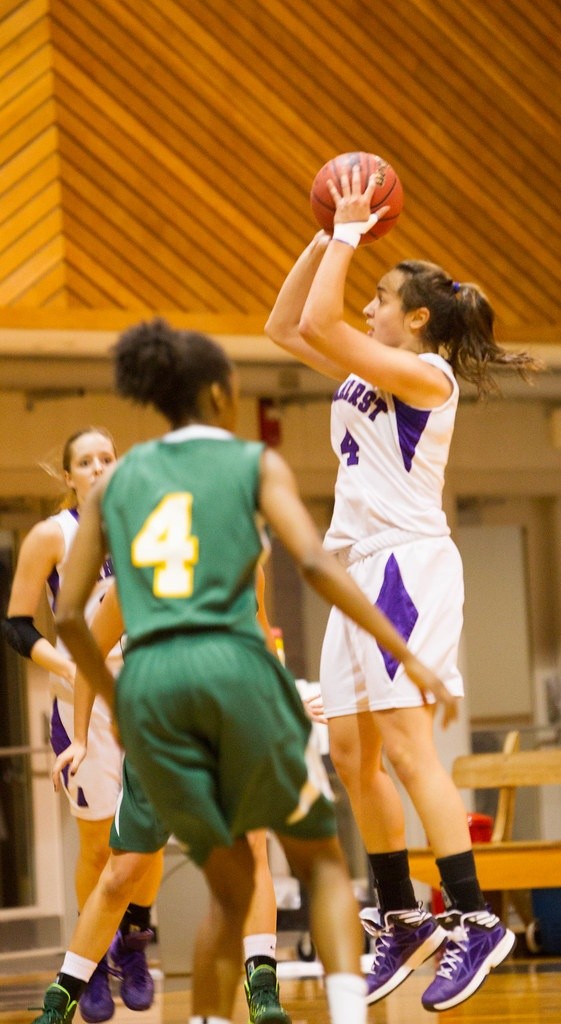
[244,964,292,1024]
[359,906,448,1007]
[108,929,154,1012]
[421,909,516,1013]
[79,956,114,1024]
[27,982,77,1024]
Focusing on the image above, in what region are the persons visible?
[2,425,168,1023]
[50,317,461,1024]
[263,165,539,1012]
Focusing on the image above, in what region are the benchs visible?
[402,731,561,926]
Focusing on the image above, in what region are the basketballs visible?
[311,149,403,245]
